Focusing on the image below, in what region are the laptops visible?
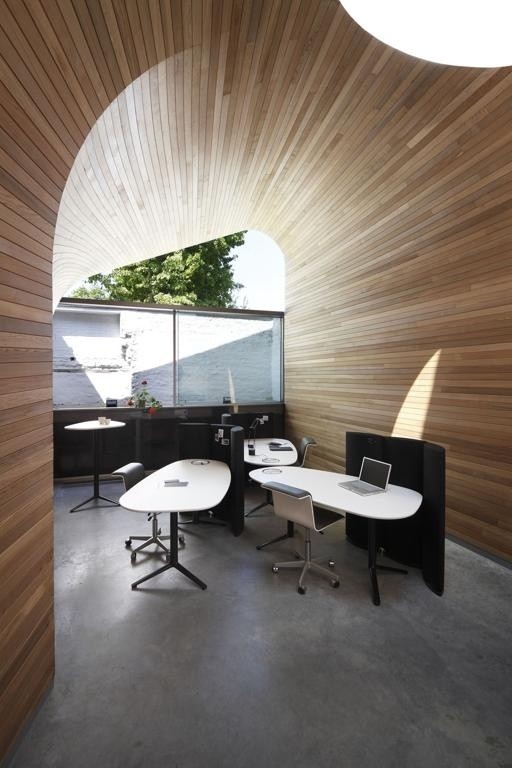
[337,456,393,497]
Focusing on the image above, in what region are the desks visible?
[118,456,231,591]
[63,418,128,512]
[248,468,426,607]
[243,436,300,519]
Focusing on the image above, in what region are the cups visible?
[98,416,111,425]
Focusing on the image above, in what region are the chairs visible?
[112,462,185,563]
[295,436,317,467]
[261,480,346,596]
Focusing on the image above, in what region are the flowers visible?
[127,379,162,415]
